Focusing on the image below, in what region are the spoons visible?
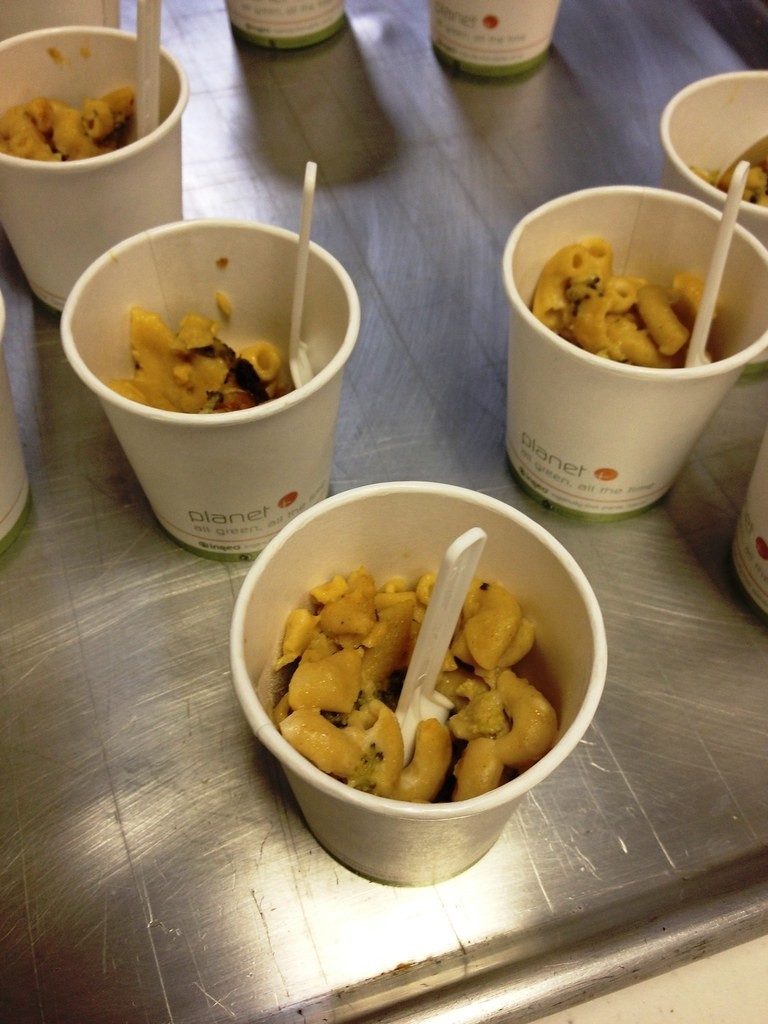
[286,161,317,391]
[389,527,487,769]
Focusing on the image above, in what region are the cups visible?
[493,185,767,523]
[60,220,363,559]
[658,64,768,237]
[0,24,190,313]
[725,419,768,632]
[225,477,608,889]
[0,292,37,565]
[427,0,560,80]
[225,0,347,51]
